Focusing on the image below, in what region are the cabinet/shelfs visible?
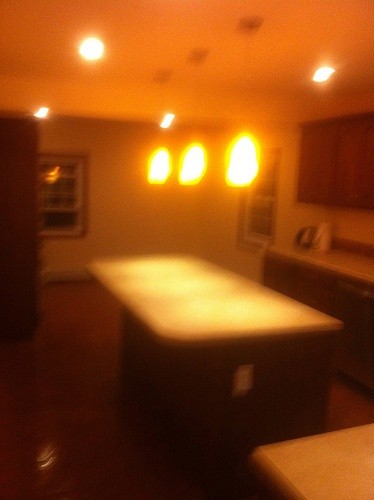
[294,105,374,209]
[262,239,374,396]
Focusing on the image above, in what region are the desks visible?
[81,252,346,495]
[248,414,374,499]
